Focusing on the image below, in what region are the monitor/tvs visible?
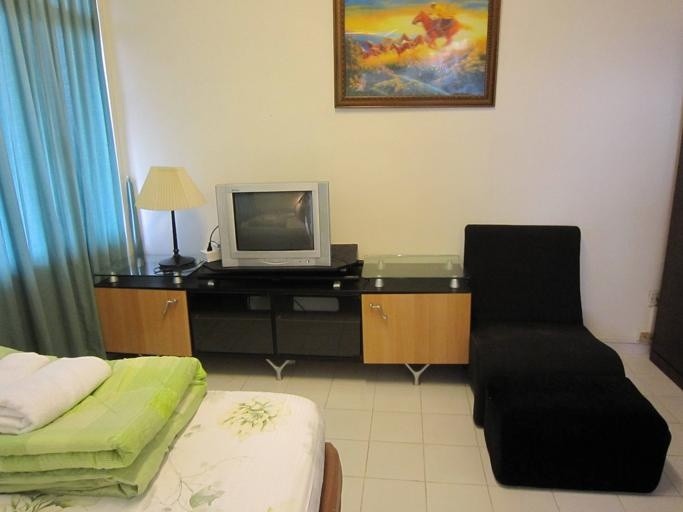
[215,180,331,267]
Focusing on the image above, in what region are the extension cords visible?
[200,242,221,263]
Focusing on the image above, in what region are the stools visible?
[483,375,673,493]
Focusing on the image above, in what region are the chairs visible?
[464,225,671,426]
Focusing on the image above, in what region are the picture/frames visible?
[333,0,502,107]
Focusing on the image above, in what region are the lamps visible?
[133,166,207,269]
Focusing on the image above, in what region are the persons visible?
[428,2,455,30]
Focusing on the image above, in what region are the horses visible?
[412,10,471,51]
[363,33,424,59]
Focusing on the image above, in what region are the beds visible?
[1,344,343,512]
